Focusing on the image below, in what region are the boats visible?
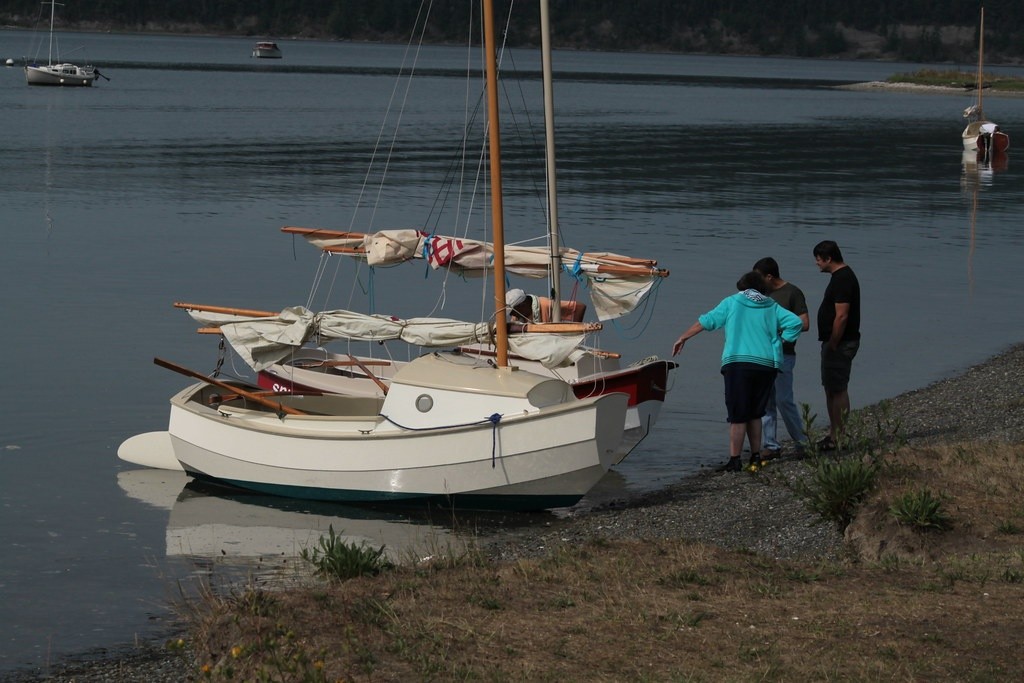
[254,40,283,59]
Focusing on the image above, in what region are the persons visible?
[813,240,862,450]
[672,271,804,471]
[979,123,1000,150]
[753,256,810,458]
[980,152,994,187]
[505,288,587,323]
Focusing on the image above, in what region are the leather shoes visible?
[714,453,761,473]
[761,446,810,461]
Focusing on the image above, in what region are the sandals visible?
[813,437,847,451]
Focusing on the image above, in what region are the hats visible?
[505,289,526,315]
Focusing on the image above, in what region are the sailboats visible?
[960,4,1011,157]
[177,5,677,471]
[24,1,110,88]
[165,3,631,510]
